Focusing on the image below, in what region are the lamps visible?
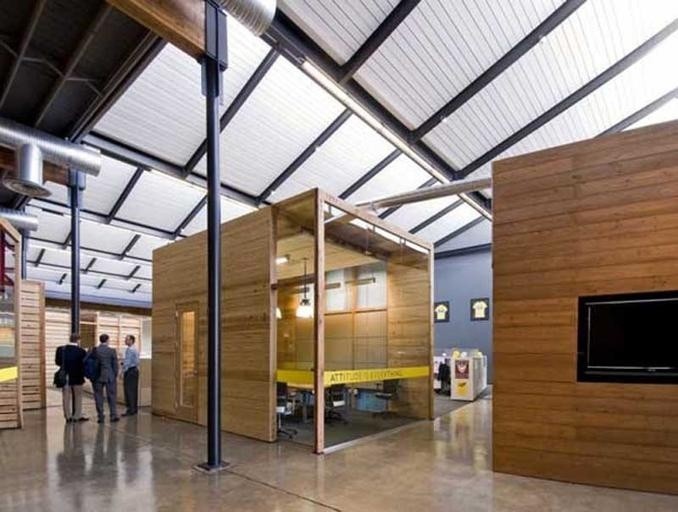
[276,254,314,320]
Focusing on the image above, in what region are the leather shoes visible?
[66,418,89,423]
[97,410,138,423]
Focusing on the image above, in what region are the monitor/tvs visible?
[586,298,677,371]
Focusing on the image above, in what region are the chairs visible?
[276,379,400,441]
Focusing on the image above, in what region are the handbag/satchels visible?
[54,368,66,388]
[84,347,101,378]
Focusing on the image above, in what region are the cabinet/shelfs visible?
[433,354,488,401]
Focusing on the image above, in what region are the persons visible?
[120,334,139,418]
[55,334,91,423]
[90,334,120,424]
